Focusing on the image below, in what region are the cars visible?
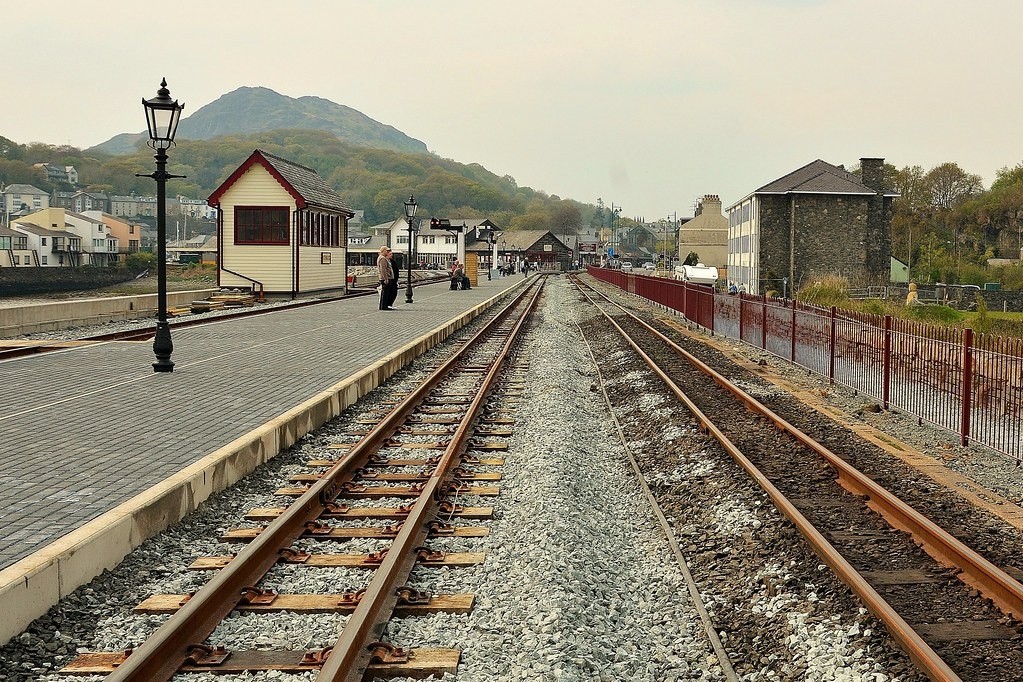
[621,262,633,273]
[645,262,656,271]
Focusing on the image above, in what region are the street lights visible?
[511,244,515,274]
[403,192,419,303]
[486,233,493,280]
[502,240,507,276]
[518,247,521,273]
[610,202,623,250]
[134,75,186,370]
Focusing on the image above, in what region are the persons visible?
[728,283,737,293]
[453,264,469,290]
[385,249,399,310]
[451,260,472,290]
[574,259,580,270]
[738,282,746,293]
[498,255,538,278]
[376,246,395,311]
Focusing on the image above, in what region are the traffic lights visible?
[430,217,439,228]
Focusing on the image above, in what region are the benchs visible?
[448,271,463,291]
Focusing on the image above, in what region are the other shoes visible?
[384,307,391,310]
